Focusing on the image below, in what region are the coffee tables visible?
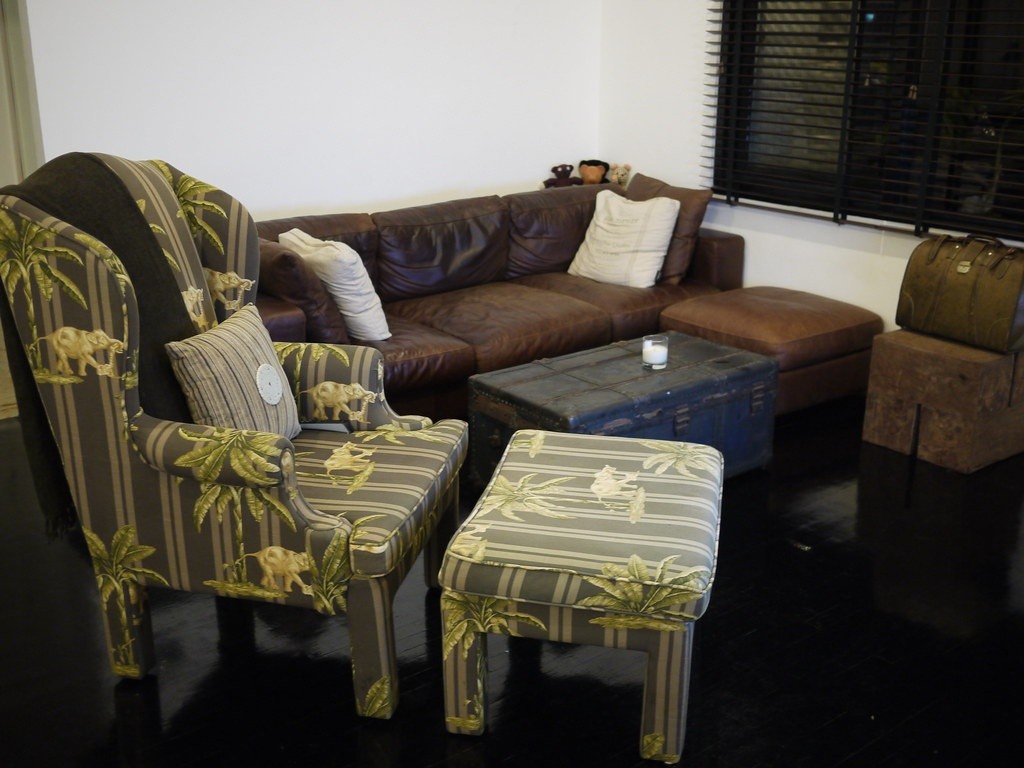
[470,329,779,482]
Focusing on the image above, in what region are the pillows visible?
[621,174,712,289]
[566,190,680,290]
[278,227,392,340]
[163,302,301,442]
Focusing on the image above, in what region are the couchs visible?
[0,151,469,720]
[255,182,744,426]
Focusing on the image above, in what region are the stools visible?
[435,429,725,766]
[863,330,1023,474]
[659,285,884,419]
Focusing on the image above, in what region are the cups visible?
[642,335,668,371]
